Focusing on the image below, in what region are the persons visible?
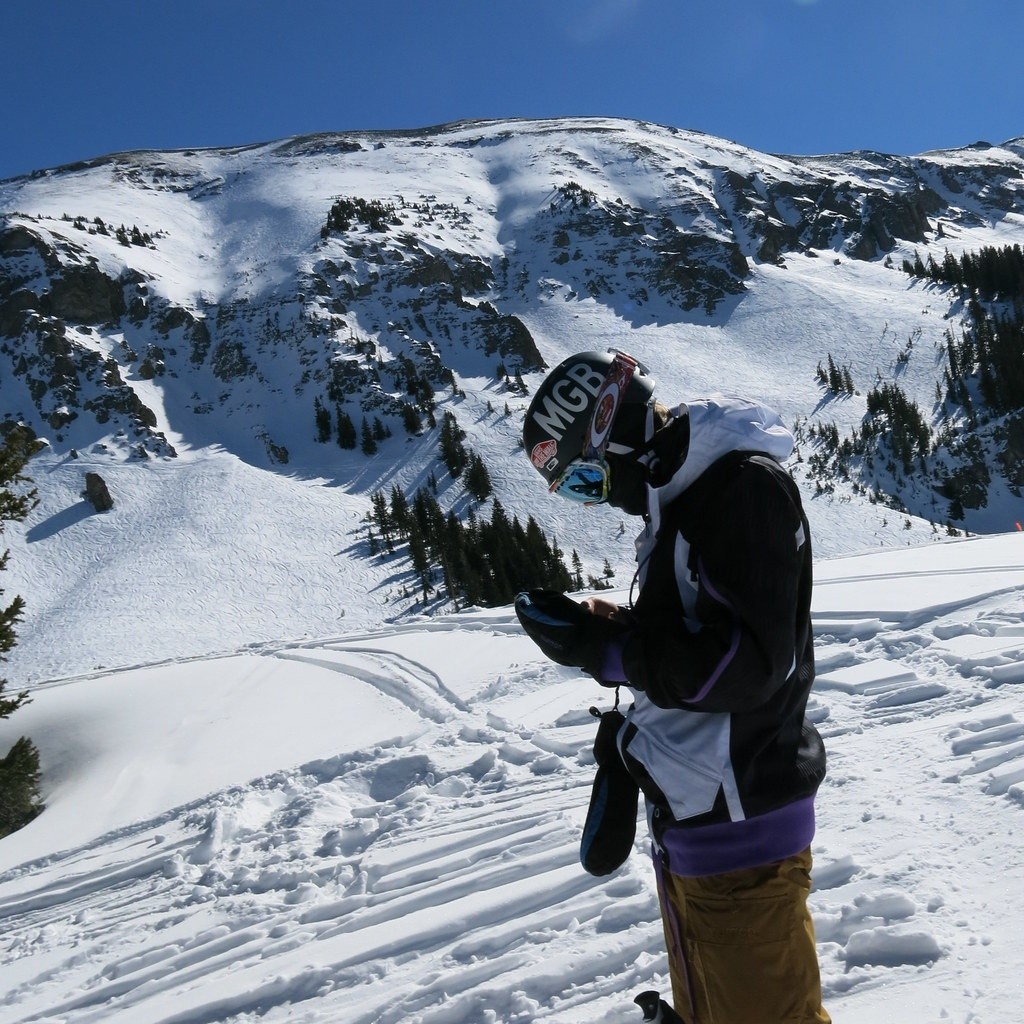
[515,350,836,1024]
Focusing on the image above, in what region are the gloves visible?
[579,704,639,877]
[515,590,626,688]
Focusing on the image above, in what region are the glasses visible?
[556,458,610,512]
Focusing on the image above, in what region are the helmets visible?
[524,348,660,515]
